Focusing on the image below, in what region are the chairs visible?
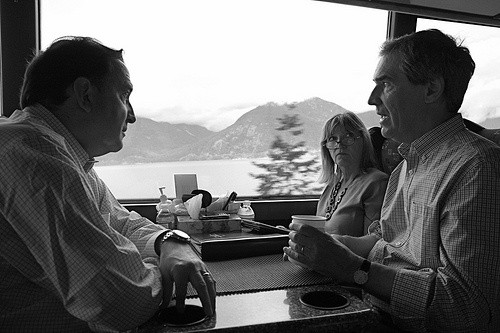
[368,118,500,177]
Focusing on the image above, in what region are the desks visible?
[127,253,380,333]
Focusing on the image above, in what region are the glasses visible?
[323,135,361,149]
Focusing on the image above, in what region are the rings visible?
[203,273,209,276]
[301,247,304,253]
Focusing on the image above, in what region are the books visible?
[241,219,290,235]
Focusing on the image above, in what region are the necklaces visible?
[325,180,347,221]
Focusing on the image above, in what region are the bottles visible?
[156,203,174,229]
[237,200,255,220]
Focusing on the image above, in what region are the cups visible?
[288,215,328,269]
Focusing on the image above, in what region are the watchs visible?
[352,259,371,289]
[161,229,191,244]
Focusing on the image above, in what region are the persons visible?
[316,110,390,237]
[283,28,500,333]
[0,35,217,333]
